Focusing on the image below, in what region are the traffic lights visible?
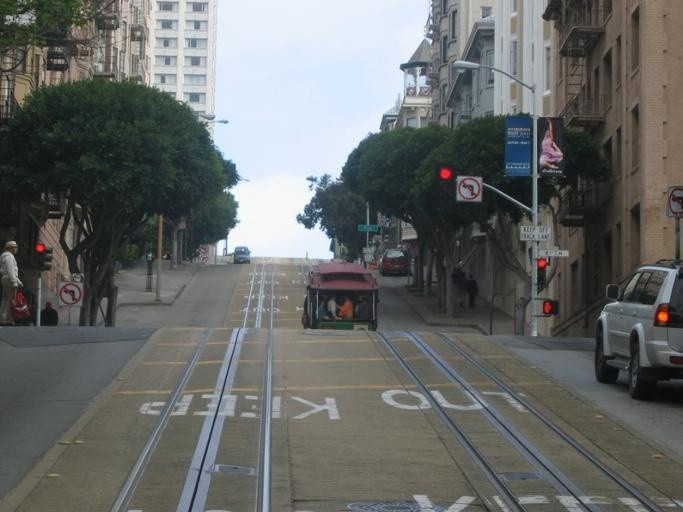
[35,244,44,268]
[437,164,454,181]
[543,300,552,315]
[45,248,53,271]
[538,258,547,270]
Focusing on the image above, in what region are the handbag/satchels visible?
[10,286,31,321]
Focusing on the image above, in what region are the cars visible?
[232,246,250,264]
[379,251,410,277]
[595,259,683,399]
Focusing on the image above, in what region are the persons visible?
[455,271,468,308]
[466,273,480,307]
[313,294,372,320]
[538,117,563,172]
[40,301,59,326]
[0,240,26,329]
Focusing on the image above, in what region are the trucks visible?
[301,262,380,331]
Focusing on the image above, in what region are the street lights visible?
[452,61,538,337]
[192,114,228,124]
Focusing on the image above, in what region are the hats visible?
[4,240,19,249]
